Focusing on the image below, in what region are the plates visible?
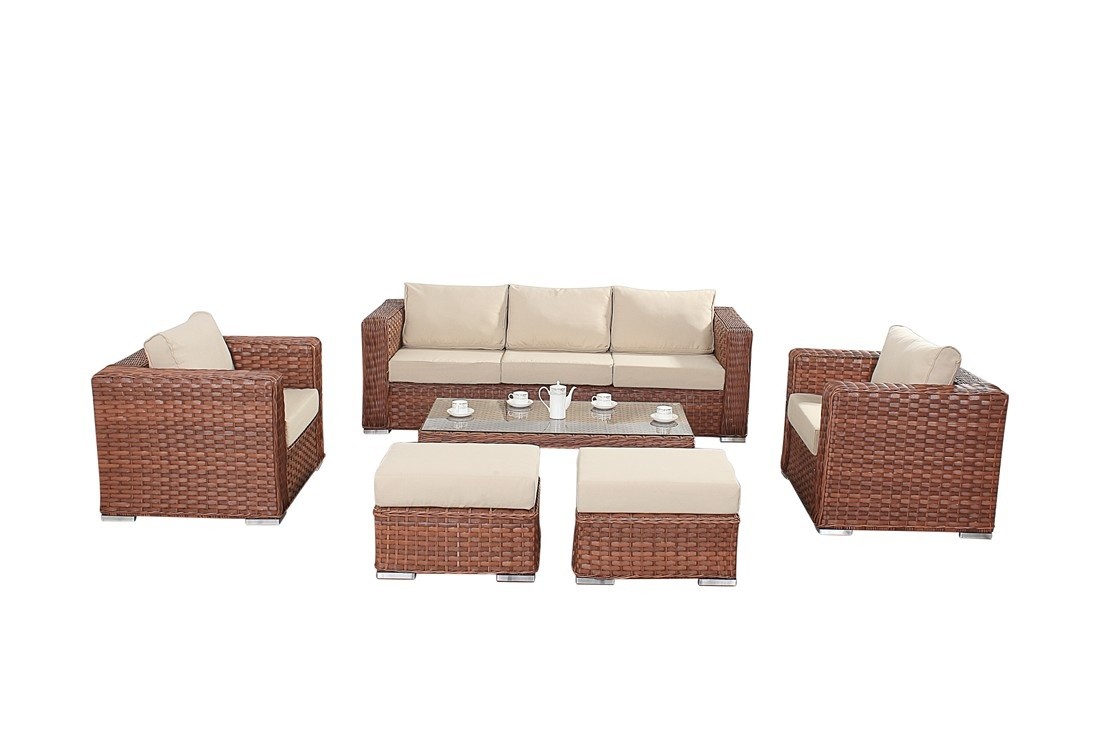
[447,408,474,417]
[591,401,618,410]
[651,413,678,422]
[591,408,615,414]
[446,416,474,422]
[650,420,678,427]
[506,399,534,408]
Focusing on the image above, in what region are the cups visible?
[657,406,672,420]
[592,393,611,406]
[508,391,528,405]
[596,415,611,421]
[452,400,468,415]
[656,427,671,435]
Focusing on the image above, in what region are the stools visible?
[372,442,541,583]
[572,445,741,587]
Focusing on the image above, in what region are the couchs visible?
[780,324,1009,540]
[91,310,326,527]
[362,282,754,444]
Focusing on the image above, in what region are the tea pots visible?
[539,381,577,420]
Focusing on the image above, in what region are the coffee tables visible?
[418,398,696,449]
